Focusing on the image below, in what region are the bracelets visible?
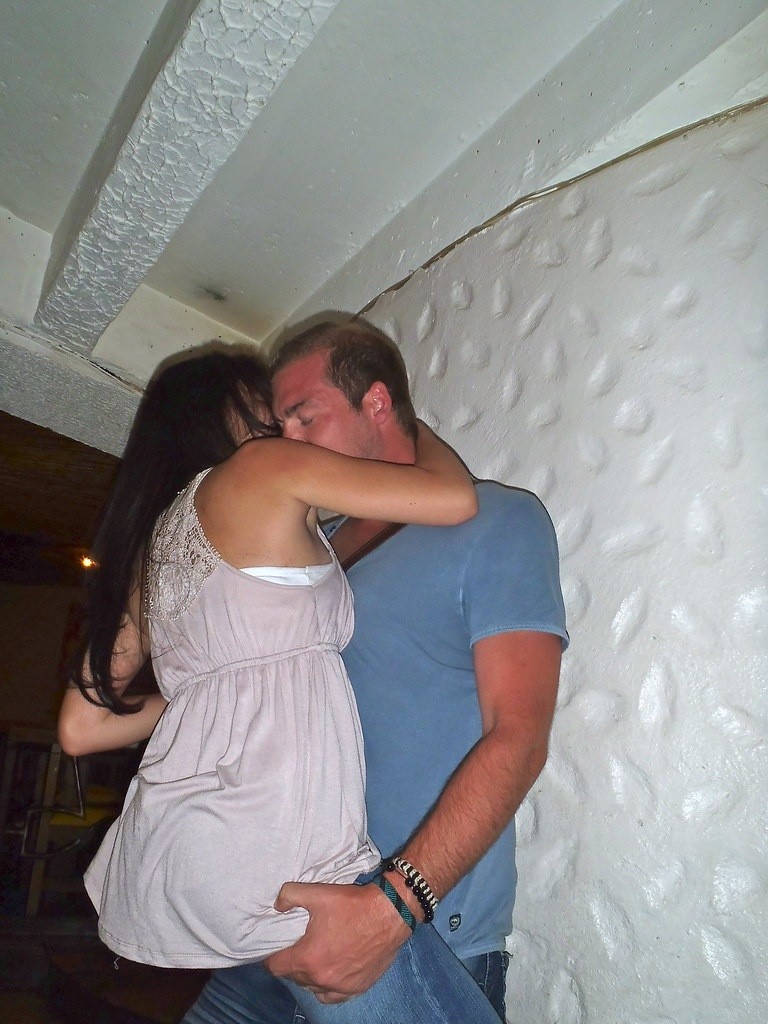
[371,854,441,933]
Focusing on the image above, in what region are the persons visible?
[177,317,567,1024]
[61,341,503,1024]
